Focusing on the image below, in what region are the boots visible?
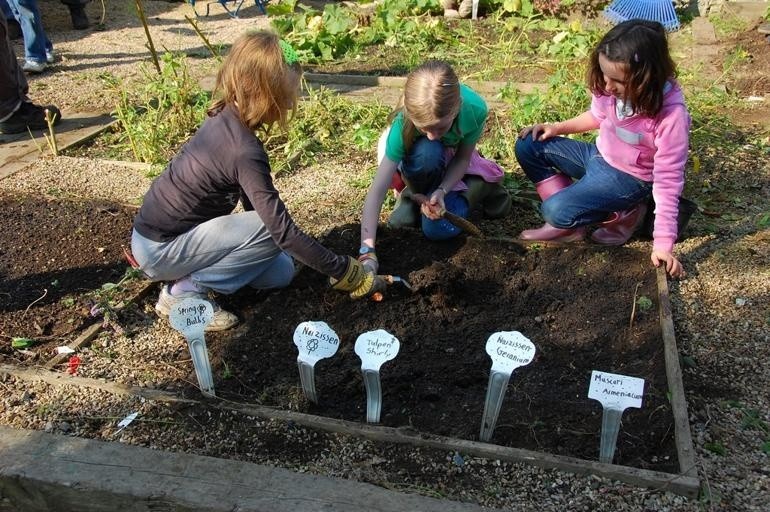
[519,172,589,242]
[592,201,647,245]
[390,172,440,228]
[459,172,512,218]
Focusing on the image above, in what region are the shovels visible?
[375,274,425,293]
[403,189,482,237]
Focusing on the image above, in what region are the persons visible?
[132,29,394,330]
[361,61,511,272]
[9,0,55,71]
[515,17,690,277]
[0,7,60,133]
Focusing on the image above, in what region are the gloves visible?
[330,256,389,301]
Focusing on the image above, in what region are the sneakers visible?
[70,8,87,29]
[155,285,240,331]
[46,49,55,64]
[5,22,21,39]
[22,61,48,72]
[0,103,61,133]
